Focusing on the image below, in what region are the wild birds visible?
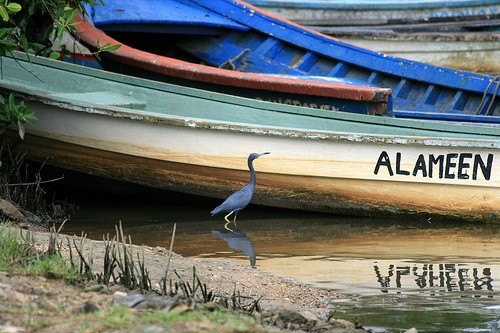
[209,152,271,223]
[210,223,261,269]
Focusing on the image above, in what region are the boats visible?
[1,47,499,222]
[30,1,498,123]
[257,0,500,76]
